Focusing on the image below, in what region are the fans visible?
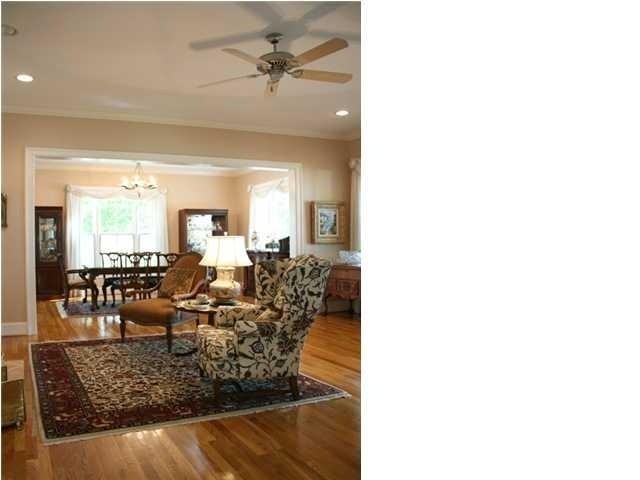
[193,31,355,103]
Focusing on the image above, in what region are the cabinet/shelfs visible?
[32,204,66,299]
[1,358,27,431]
[241,247,289,298]
[177,207,232,295]
[323,263,361,320]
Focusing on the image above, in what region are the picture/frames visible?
[309,198,347,246]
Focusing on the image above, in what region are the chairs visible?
[114,249,209,352]
[56,248,219,313]
[193,252,334,408]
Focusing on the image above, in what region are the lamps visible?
[120,162,161,200]
[195,230,255,308]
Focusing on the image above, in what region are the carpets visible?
[52,297,137,320]
[26,327,353,450]
